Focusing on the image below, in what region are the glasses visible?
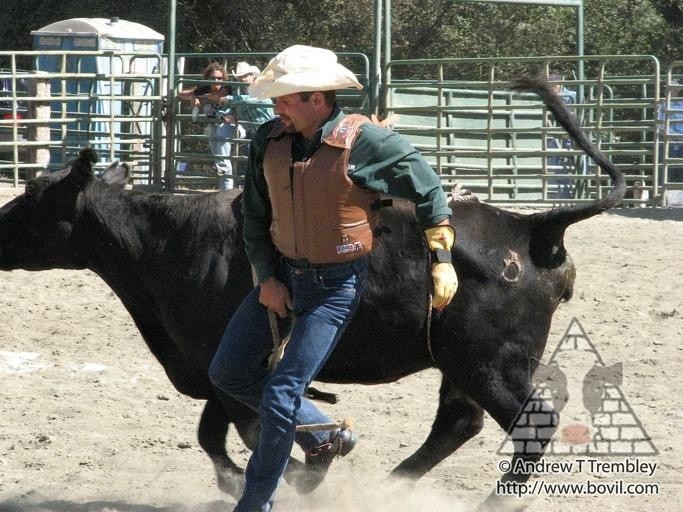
[213,77,223,80]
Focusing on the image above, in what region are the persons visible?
[208,45,459,512]
[547,73,591,199]
[177,62,276,193]
[656,80,683,182]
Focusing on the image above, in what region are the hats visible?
[232,62,261,79]
[247,44,364,99]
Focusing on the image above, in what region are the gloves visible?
[423,224,459,308]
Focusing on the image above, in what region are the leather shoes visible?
[296,426,357,496]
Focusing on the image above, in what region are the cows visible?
[0,67,629,512]
